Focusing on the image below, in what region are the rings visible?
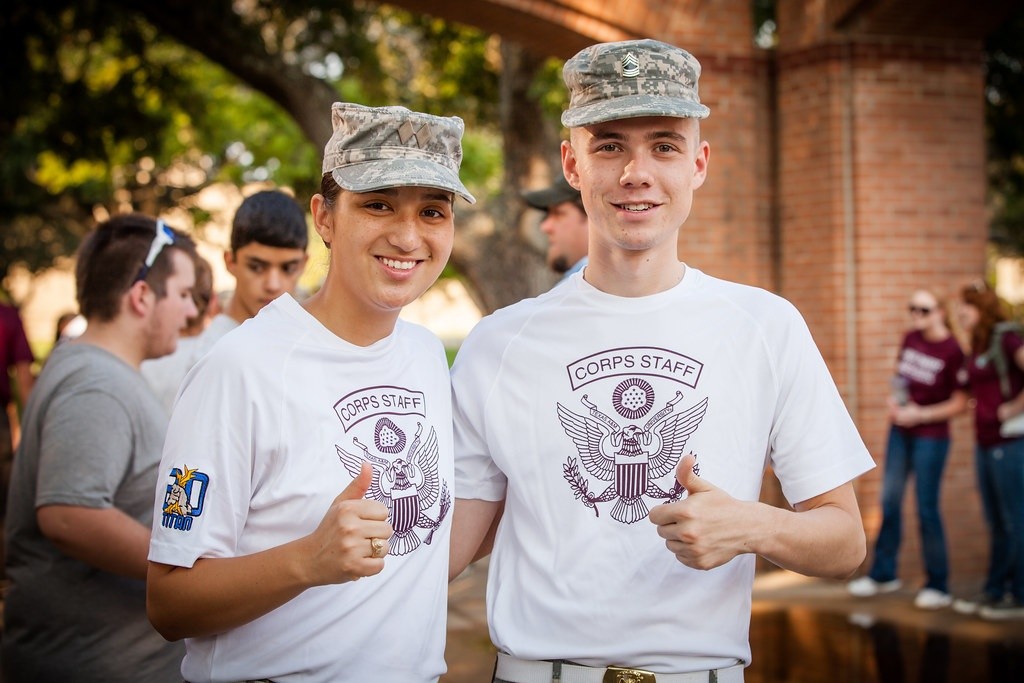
[371,537,384,558]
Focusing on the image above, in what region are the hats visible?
[321,101,477,205]
[523,176,581,212]
[560,39,711,128]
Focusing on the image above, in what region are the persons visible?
[449,36,877,683]
[56,312,78,341]
[157,190,310,409]
[145,101,477,683]
[518,175,589,286]
[846,282,969,609]
[0,305,34,533]
[139,251,213,411]
[0,213,201,683]
[954,277,1024,623]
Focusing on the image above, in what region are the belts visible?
[494,651,747,683]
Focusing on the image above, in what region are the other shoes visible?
[953,597,992,616]
[914,587,953,610]
[976,603,1024,620]
[846,575,903,597]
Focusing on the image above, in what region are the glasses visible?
[907,304,940,315]
[131,219,176,287]
